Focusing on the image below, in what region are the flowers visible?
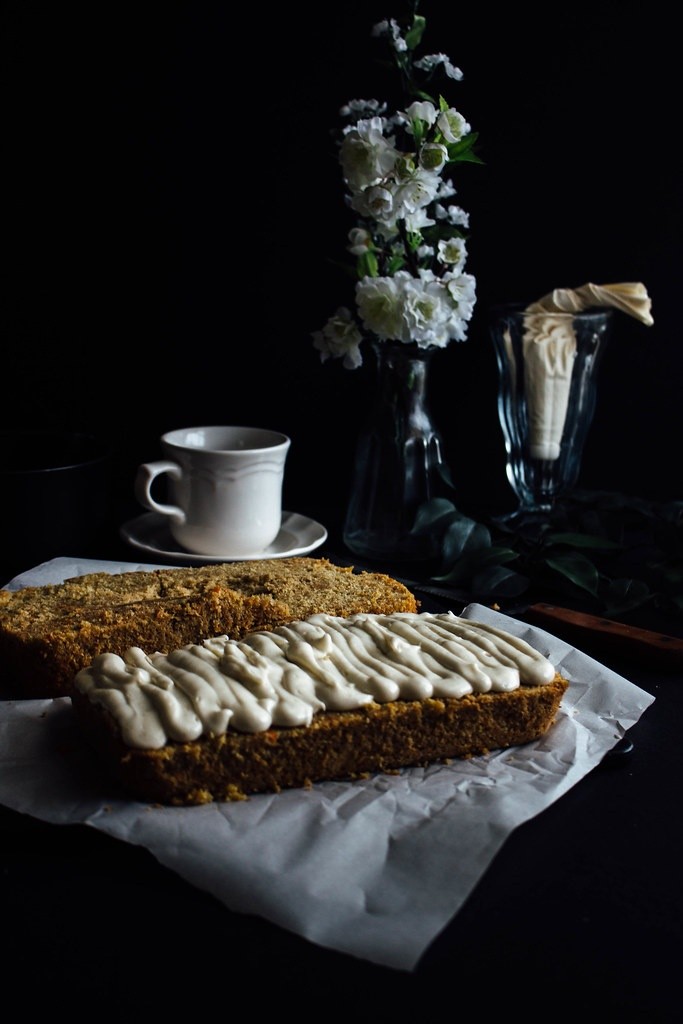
[307,14,490,371]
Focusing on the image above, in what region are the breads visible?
[66,611,569,807]
[0,555,419,696]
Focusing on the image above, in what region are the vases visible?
[343,337,445,579]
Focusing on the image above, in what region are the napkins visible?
[521,281,657,460]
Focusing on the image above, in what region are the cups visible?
[492,308,611,540]
[137,425,291,556]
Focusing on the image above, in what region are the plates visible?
[122,512,329,562]
